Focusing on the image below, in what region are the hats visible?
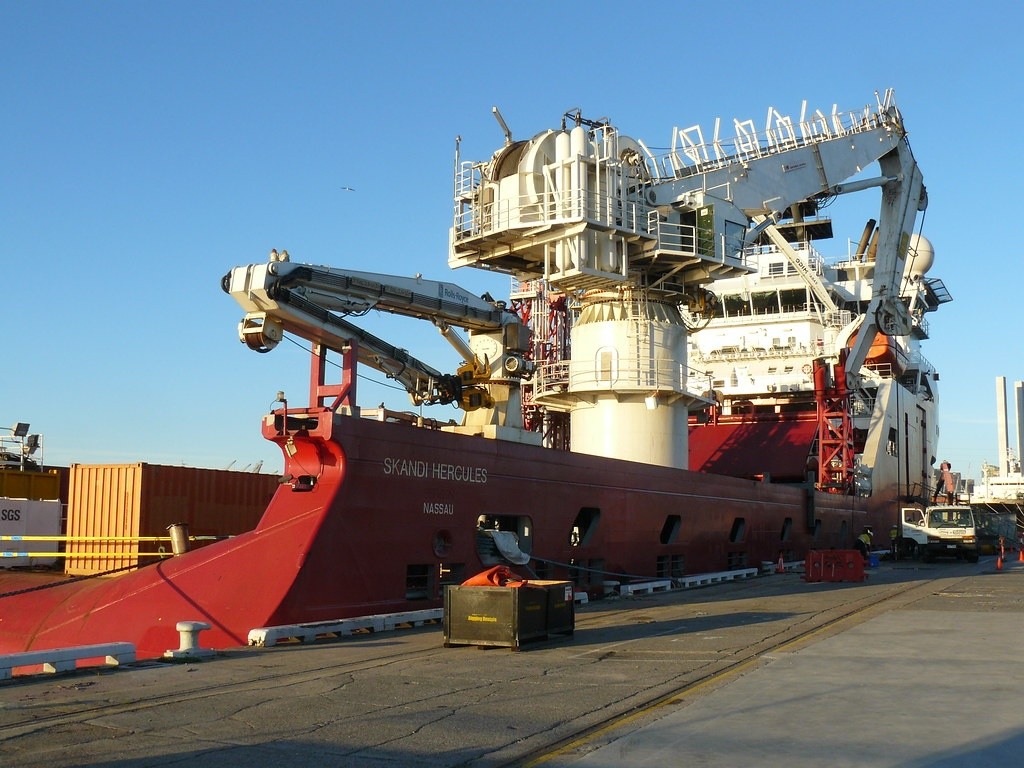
[906,517,910,521]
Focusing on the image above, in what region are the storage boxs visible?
[0,463,284,577]
[442,578,576,650]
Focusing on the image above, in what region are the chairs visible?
[957,519,969,525]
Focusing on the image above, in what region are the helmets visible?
[869,532,873,537]
[893,525,897,527]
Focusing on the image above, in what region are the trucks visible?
[900,462,981,563]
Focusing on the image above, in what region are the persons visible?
[856,529,874,569]
[888,525,897,557]
[954,512,961,524]
[1016,529,1024,552]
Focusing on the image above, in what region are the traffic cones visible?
[774,558,785,573]
[995,556,1003,571]
[1017,550,1024,562]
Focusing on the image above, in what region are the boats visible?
[0,84,1024,679]
